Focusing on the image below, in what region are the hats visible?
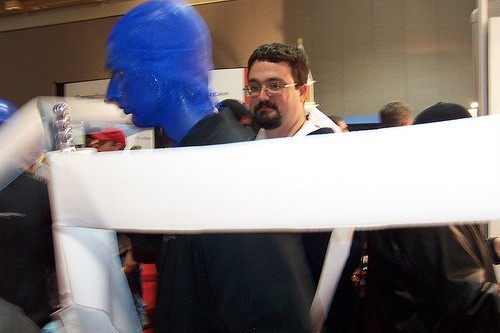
[86,127,125,147]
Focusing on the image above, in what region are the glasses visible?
[243,80,307,97]
